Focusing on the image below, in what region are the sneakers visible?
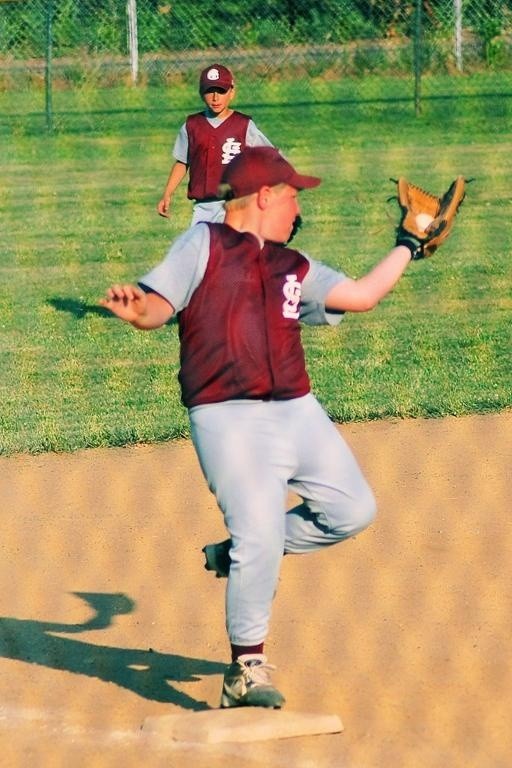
[220,653,288,711]
[203,537,235,580]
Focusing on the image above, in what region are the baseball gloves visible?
[387,176,476,260]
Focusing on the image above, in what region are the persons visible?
[158,60,280,227]
[102,143,468,707]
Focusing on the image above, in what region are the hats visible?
[219,143,322,201]
[198,63,233,95]
